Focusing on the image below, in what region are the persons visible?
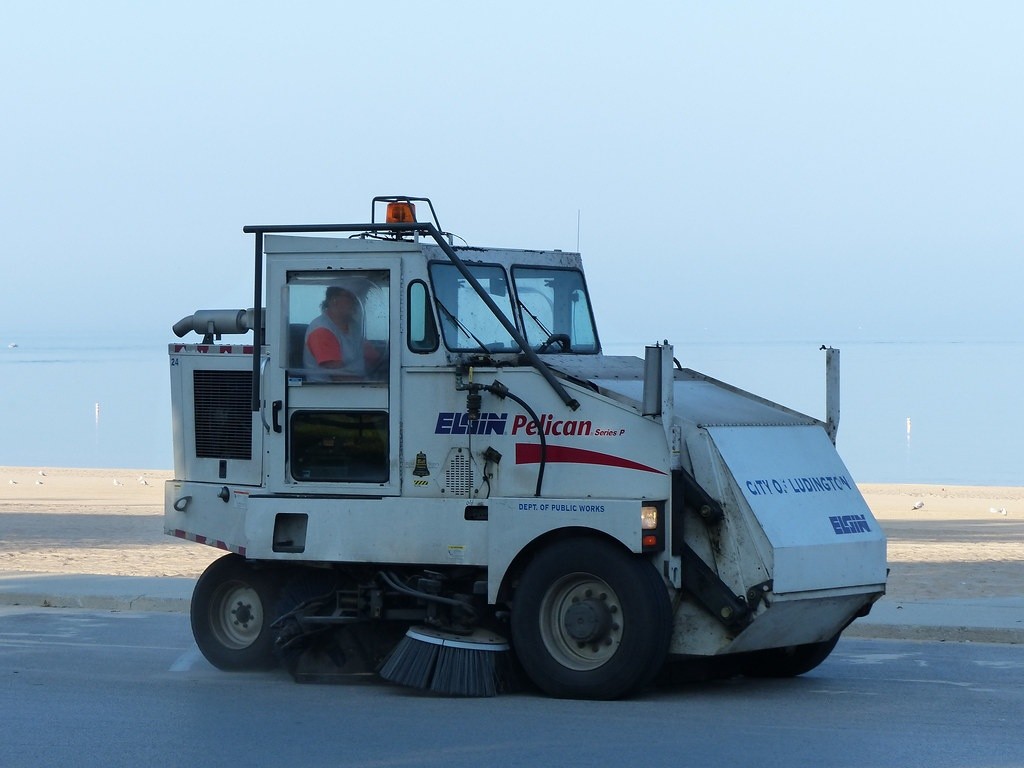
[303,286,388,382]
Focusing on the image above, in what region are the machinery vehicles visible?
[164,194,889,700]
